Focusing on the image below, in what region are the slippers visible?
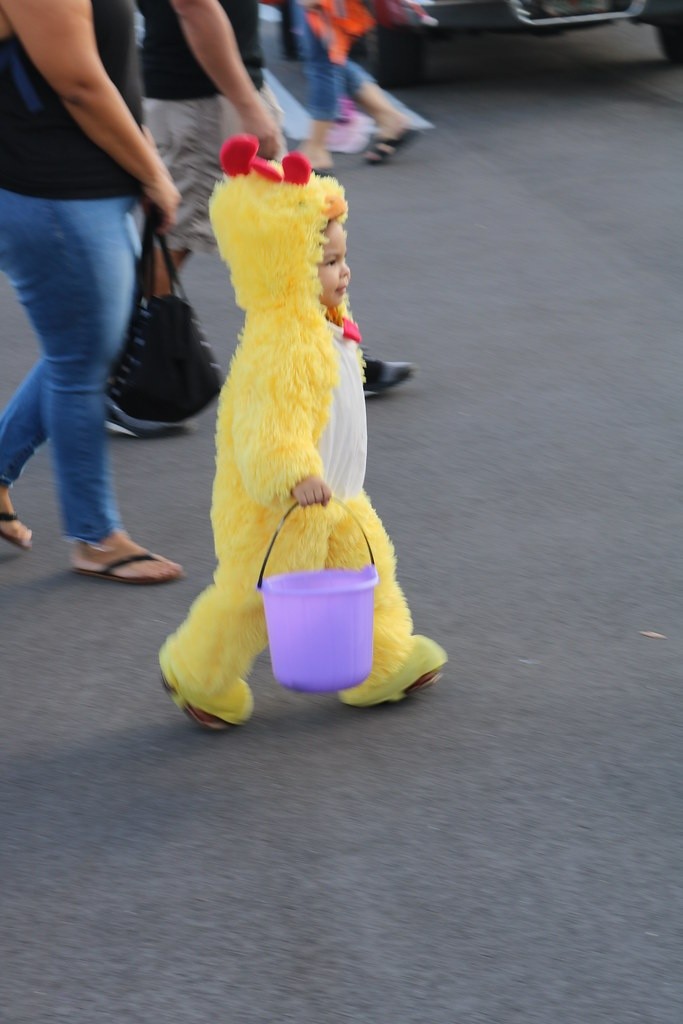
[0,510,37,551]
[71,542,180,584]
[293,149,338,177]
[360,127,419,166]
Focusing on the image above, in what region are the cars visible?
[280,0,683,85]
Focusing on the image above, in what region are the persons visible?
[277,0,436,173]
[0,0,194,587]
[160,132,452,736]
[105,0,418,440]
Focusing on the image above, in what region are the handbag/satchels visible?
[106,206,227,417]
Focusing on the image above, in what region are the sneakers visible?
[359,356,415,394]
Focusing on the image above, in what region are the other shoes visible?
[161,642,257,734]
[341,638,449,711]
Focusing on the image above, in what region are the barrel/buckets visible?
[257,495,379,696]
